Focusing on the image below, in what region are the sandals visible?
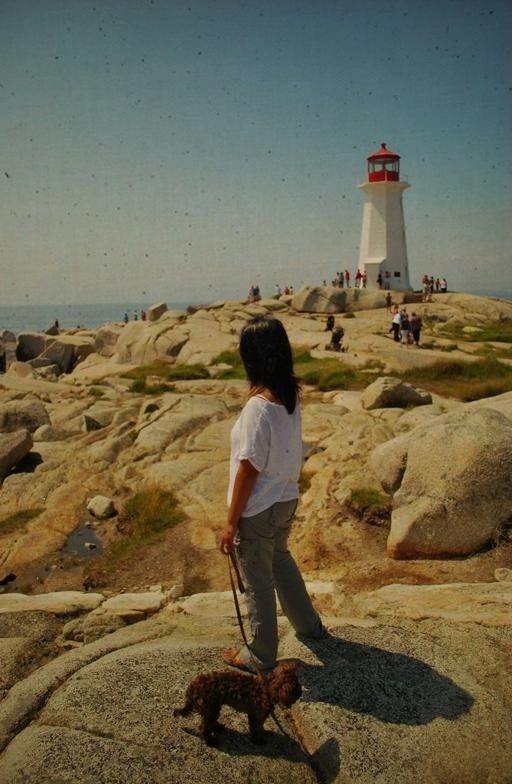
[221,648,262,675]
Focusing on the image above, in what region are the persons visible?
[55,318,59,328]
[322,269,367,289]
[327,312,335,331]
[217,315,327,671]
[123,308,146,324]
[247,284,294,304]
[376,270,447,345]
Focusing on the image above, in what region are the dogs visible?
[173,661,304,741]
